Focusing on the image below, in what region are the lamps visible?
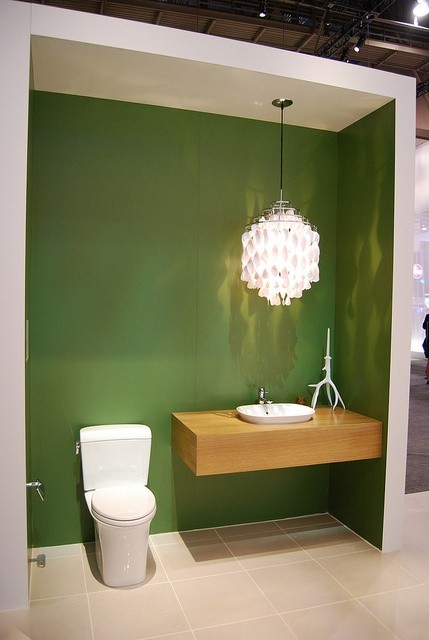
[239,98,321,308]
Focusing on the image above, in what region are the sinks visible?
[236,403,315,424]
[258,387,273,404]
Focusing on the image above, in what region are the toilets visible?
[79,424,157,587]
